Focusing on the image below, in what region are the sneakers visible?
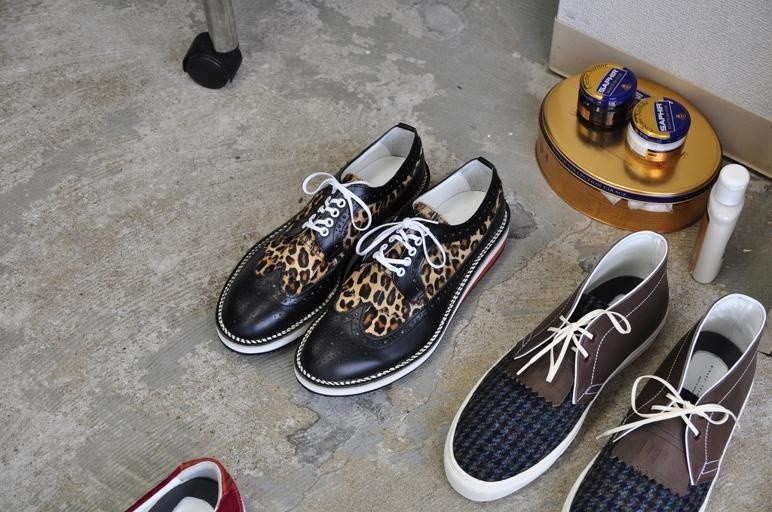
[123,457,245,511]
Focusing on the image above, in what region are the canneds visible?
[576,63,637,148]
[623,97,691,185]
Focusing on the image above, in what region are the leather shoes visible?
[442,229,670,502]
[216,123,430,355]
[293,156,511,397]
[562,292,766,512]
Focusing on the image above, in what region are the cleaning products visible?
[690,163,749,284]
